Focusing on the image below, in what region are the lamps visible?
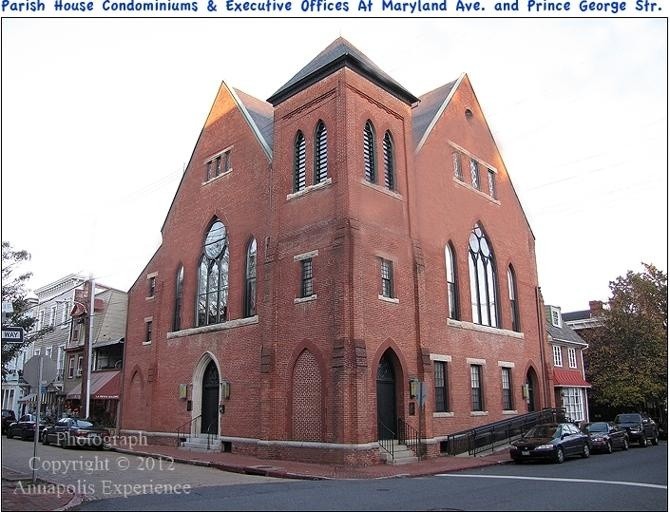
[221,382,229,400]
[409,380,416,397]
[180,384,186,399]
[522,384,529,398]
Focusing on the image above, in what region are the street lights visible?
[54,298,94,421]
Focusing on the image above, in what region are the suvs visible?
[614,412,661,447]
[1,408,17,433]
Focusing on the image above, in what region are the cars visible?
[509,423,592,466]
[7,413,50,441]
[581,420,630,453]
[41,417,110,450]
